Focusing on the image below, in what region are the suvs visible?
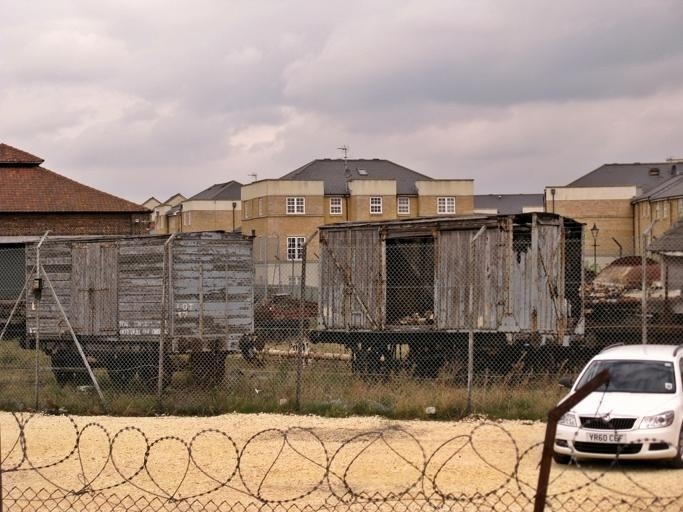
[548,341,683,468]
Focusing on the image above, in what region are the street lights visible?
[588,222,600,272]
